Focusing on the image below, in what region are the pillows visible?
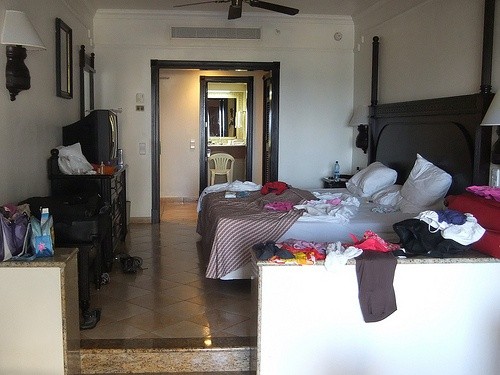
[401,153,453,209]
[345,160,398,198]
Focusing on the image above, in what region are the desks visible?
[65,231,105,311]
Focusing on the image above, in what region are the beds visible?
[194,0,496,284]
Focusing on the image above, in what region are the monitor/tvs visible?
[62,109,119,165]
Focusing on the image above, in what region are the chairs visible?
[207,152,236,186]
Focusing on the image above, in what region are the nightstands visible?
[320,174,355,188]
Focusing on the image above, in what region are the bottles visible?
[333,161,340,182]
[97,161,105,175]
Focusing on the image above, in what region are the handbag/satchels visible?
[56,142,93,175]
[392,219,475,258]
[0,203,31,262]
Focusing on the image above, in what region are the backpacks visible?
[12,214,55,260]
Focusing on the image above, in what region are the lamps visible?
[479,87,500,166]
[0,9,48,103]
[238,110,246,128]
[348,107,368,154]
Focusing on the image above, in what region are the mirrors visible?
[206,97,238,140]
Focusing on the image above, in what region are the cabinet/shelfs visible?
[43,163,129,264]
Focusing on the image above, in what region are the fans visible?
[172,0,300,21]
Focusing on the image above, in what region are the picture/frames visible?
[54,15,74,101]
[79,43,97,120]
